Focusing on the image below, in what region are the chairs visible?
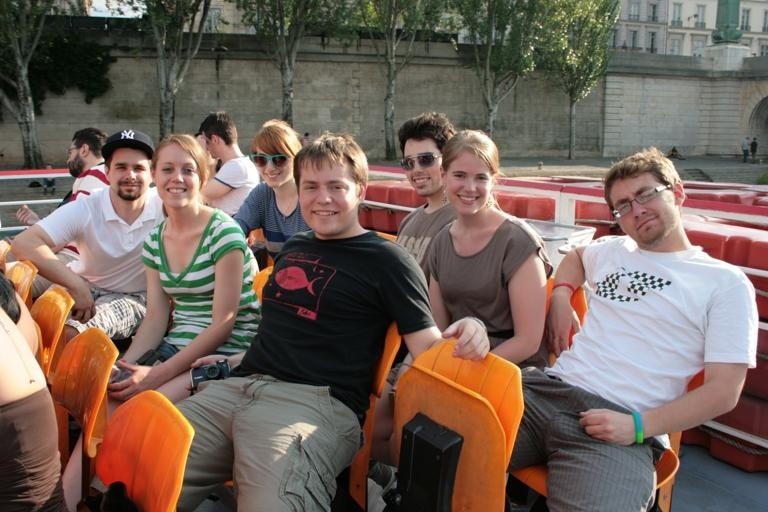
[344,320,403,512]
[384,337,525,511]
[6,259,40,311]
[513,434,683,512]
[51,326,120,511]
[0,237,13,274]
[544,275,588,369]
[30,282,76,389]
[93,389,195,511]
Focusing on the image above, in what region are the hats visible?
[100,129,155,159]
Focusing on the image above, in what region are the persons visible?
[1,309,68,512]
[98,130,490,511]
[742,137,757,164]
[0,266,39,363]
[233,119,312,264]
[300,131,310,145]
[367,129,553,512]
[0,127,111,301]
[395,112,457,269]
[506,145,760,512]
[10,129,165,392]
[198,111,260,216]
[61,132,263,511]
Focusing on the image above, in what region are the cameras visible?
[190,358,230,389]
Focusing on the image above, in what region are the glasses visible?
[249,152,288,167]
[612,185,671,219]
[401,153,443,170]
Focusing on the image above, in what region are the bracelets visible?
[631,410,643,444]
[552,282,575,293]
[463,316,488,334]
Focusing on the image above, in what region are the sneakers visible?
[367,461,399,512]
